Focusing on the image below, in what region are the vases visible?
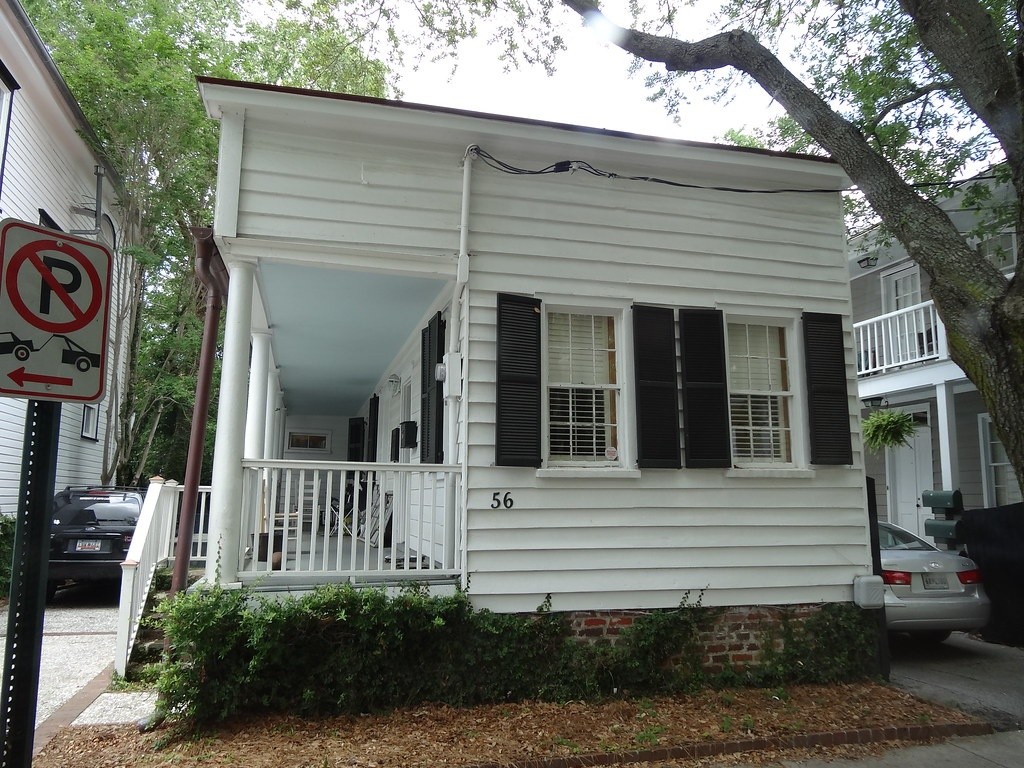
[250,532,282,562]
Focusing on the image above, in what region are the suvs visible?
[46,484,147,599]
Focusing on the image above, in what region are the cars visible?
[878,520,991,647]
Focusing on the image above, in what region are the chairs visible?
[293,479,321,532]
[918,325,938,364]
[261,478,298,552]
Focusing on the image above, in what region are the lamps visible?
[387,373,401,398]
[861,396,883,407]
[857,256,878,269]
